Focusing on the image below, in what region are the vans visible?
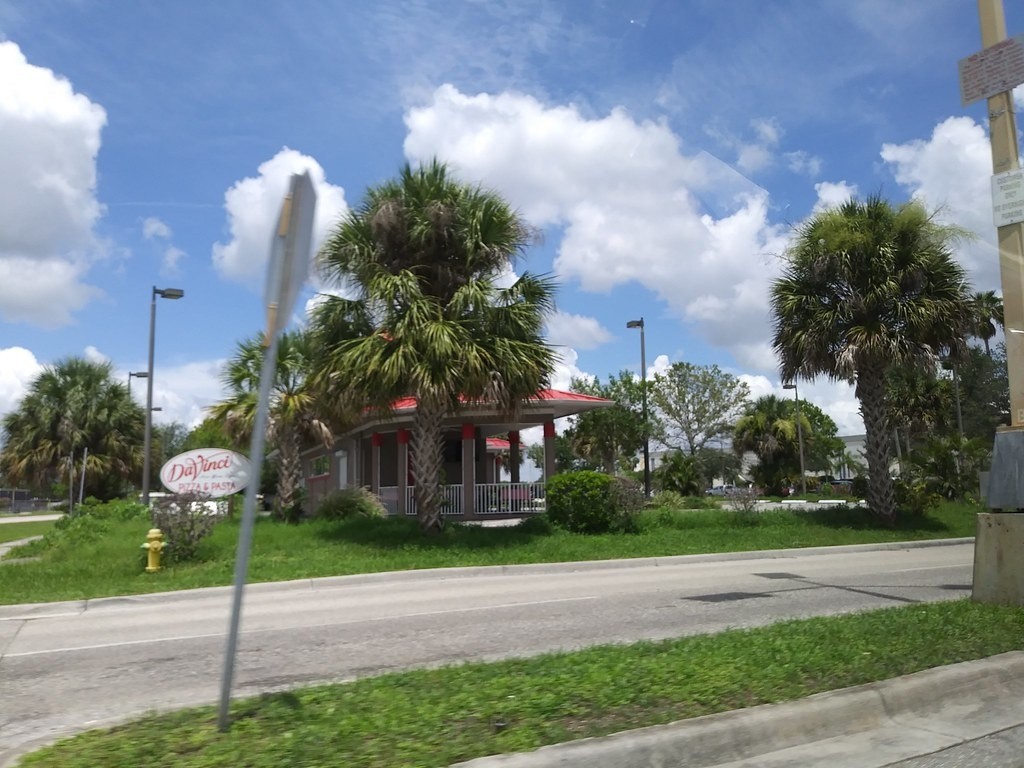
[707,484,733,498]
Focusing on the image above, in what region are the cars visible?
[811,480,853,493]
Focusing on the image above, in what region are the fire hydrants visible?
[139,527,169,574]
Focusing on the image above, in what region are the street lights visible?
[127,370,149,403]
[141,285,184,510]
[626,316,651,498]
[783,384,807,496]
